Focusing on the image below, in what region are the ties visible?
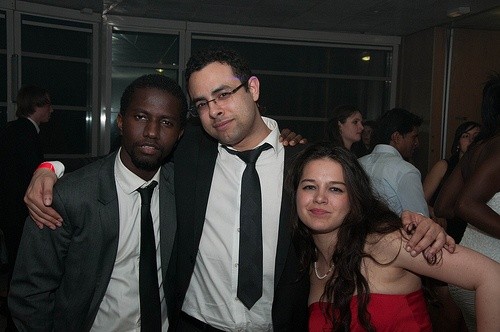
[220,142,274,310]
[136,180,163,332]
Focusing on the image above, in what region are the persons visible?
[327,105,378,156]
[422,120,482,332]
[0,83,55,294]
[435,71,500,332]
[22,44,456,332]
[358,121,377,152]
[355,108,430,218]
[8,76,307,332]
[283,143,500,332]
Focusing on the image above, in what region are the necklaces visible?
[314,247,335,279]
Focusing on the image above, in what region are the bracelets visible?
[37,163,55,173]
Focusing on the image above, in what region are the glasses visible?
[188,81,247,118]
